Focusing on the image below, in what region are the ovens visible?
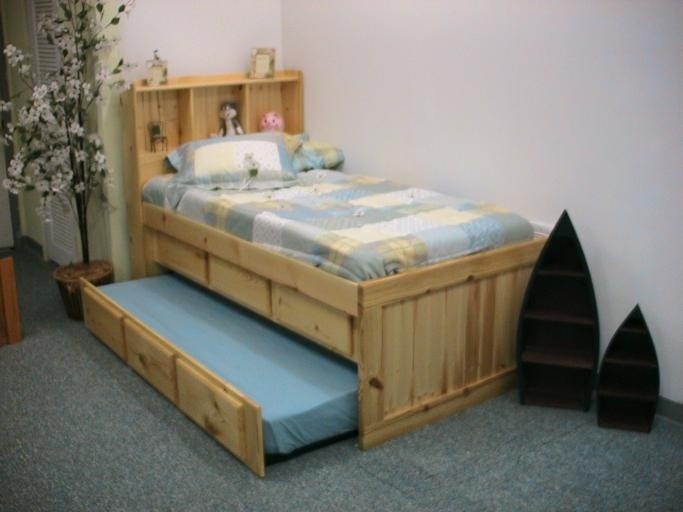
[76,68,555,475]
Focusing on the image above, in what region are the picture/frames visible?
[0,245,22,348]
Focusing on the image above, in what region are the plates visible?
[512,209,596,412]
[597,301,662,430]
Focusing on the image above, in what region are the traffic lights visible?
[163,132,297,192]
[290,133,347,174]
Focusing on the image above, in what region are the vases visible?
[0,0,165,259]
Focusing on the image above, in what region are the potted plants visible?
[248,47,278,82]
[144,58,168,87]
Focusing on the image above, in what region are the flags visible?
[53,263,114,321]
